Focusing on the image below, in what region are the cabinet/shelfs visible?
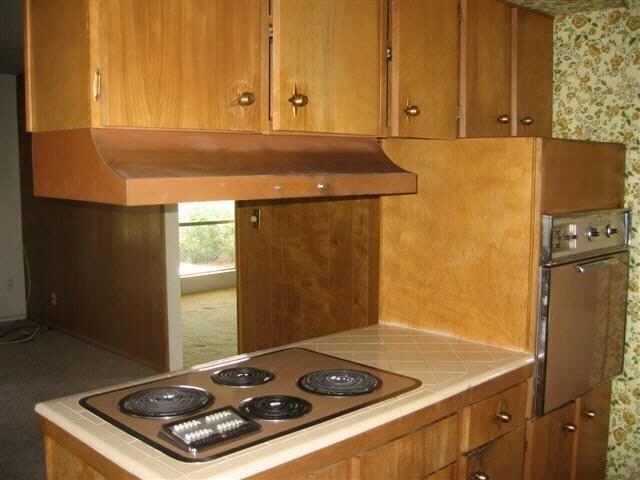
[355,392,460,480]
[243,436,354,480]
[534,377,612,479]
[459,357,534,478]
[23,2,386,143]
[460,4,557,143]
[386,2,457,141]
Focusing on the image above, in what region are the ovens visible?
[531,211,632,419]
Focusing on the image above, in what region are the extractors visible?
[30,130,417,207]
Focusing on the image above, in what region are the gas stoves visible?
[77,342,423,465]
